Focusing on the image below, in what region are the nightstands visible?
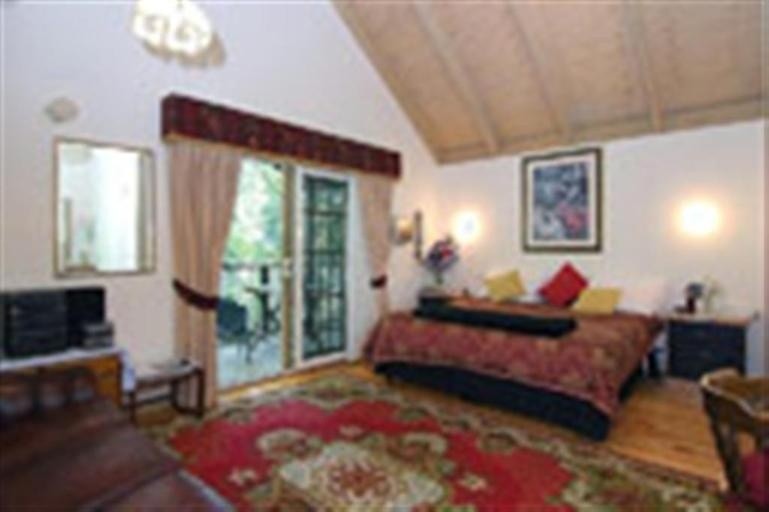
[417,289,462,306]
[663,304,759,381]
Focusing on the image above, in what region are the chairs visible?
[699,365,769,512]
[215,297,265,367]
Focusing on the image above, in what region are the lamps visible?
[131,0,212,57]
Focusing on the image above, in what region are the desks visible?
[1,347,137,478]
[129,358,208,422]
[245,280,282,342]
[1,401,215,508]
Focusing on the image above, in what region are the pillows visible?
[576,285,624,314]
[534,258,593,308]
[482,267,526,301]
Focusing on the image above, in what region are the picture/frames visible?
[520,144,602,252]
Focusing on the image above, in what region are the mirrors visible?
[52,135,158,275]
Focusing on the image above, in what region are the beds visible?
[368,290,663,438]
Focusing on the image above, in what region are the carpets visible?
[147,363,726,512]
